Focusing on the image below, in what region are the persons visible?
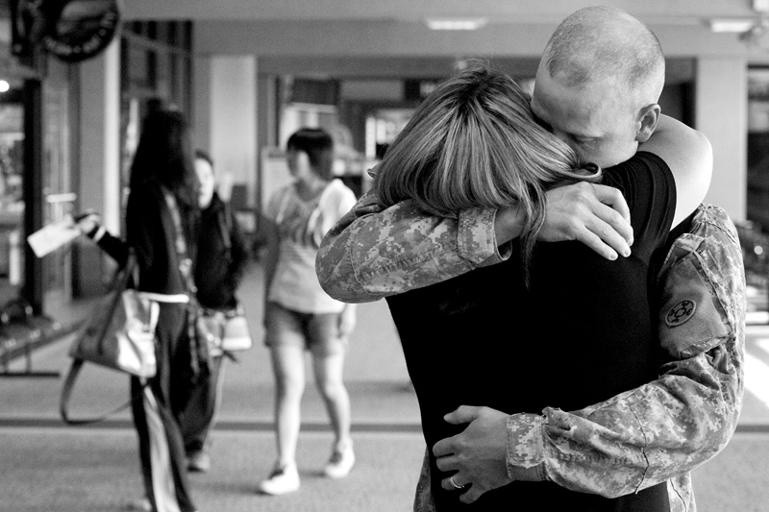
[316,5,746,512]
[125,94,202,512]
[256,126,360,496]
[377,66,713,512]
[74,146,249,471]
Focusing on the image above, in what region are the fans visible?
[23,0,118,69]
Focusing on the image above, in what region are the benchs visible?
[0,300,62,383]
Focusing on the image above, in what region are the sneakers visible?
[324,447,356,480]
[185,450,211,473]
[256,468,301,496]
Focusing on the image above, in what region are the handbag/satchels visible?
[195,313,253,358]
[67,288,159,380]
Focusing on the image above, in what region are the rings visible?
[449,476,464,489]
